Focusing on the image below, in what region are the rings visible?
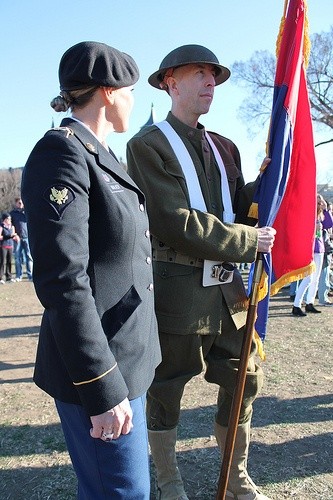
[102,433,115,441]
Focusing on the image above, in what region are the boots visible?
[214,414,271,500]
[147,425,189,500]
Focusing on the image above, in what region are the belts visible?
[152,250,203,268]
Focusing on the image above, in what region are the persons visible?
[21,41,161,500]
[0,197,33,285]
[289,194,333,315]
[127,44,272,500]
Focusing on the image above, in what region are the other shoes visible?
[289,295,295,302]
[319,302,333,306]
[7,279,16,283]
[0,280,4,284]
[15,277,22,282]
[305,304,321,313]
[316,290,333,299]
[292,307,307,316]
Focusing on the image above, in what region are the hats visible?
[2,213,10,221]
[59,41,140,91]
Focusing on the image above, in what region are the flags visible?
[243,0,317,360]
[139,108,153,128]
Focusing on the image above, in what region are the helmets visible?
[147,45,231,90]
